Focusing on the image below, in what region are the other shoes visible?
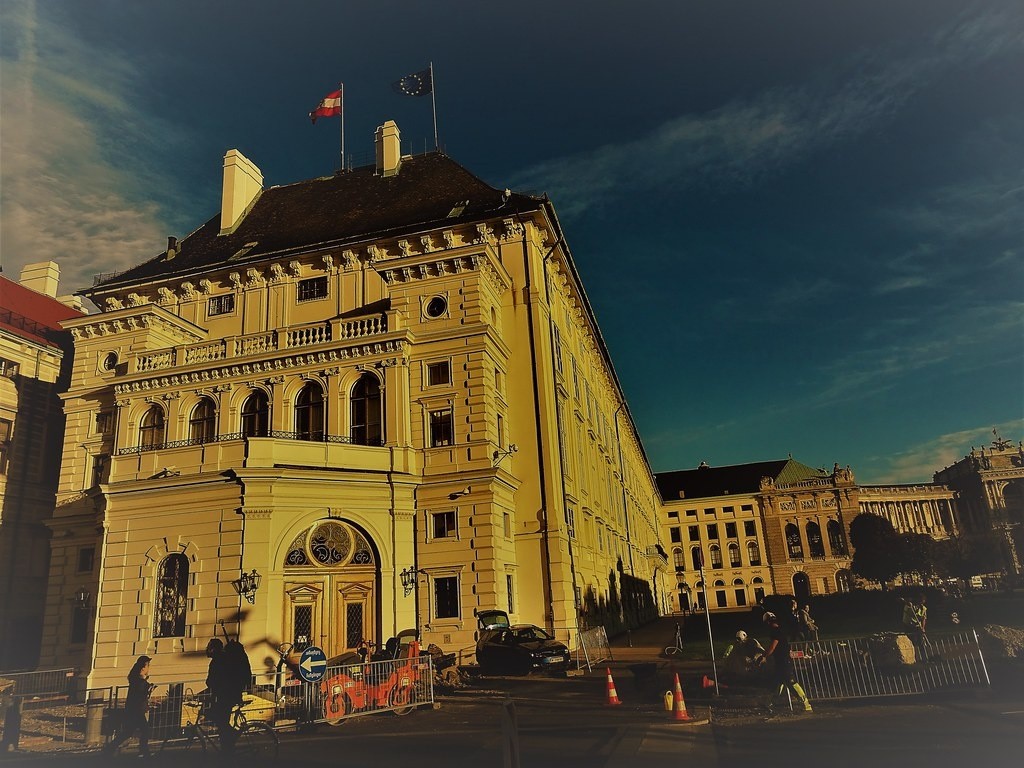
[138,751,157,758]
[822,651,830,656]
[804,655,812,659]
[812,651,819,657]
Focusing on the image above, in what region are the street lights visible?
[675,569,689,633]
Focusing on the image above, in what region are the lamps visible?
[400,565,418,598]
[239,570,263,604]
[75,585,94,617]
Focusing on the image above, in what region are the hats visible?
[137,656,152,663]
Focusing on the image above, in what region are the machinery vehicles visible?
[314,628,428,728]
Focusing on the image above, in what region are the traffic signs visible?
[298,646,327,683]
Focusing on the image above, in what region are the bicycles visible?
[158,688,282,768]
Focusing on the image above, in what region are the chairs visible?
[369,636,401,679]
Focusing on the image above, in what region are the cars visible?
[473,609,571,674]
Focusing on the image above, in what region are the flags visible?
[311,83,342,117]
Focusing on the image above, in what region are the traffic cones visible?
[672,671,694,721]
[702,673,730,690]
[603,666,623,705]
[792,648,804,658]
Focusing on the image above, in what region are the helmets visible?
[762,611,775,624]
[736,630,748,641]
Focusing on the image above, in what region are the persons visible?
[98,655,158,761]
[195,639,244,768]
[787,599,831,657]
[905,592,928,665]
[682,607,685,615]
[721,611,813,711]
[692,601,697,615]
[356,637,379,662]
